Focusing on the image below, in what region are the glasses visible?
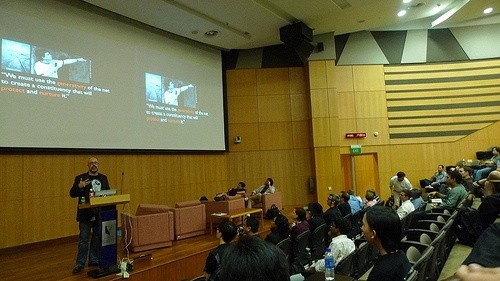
[89,162,99,165]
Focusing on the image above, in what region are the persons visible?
[245,178,276,209]
[33,49,88,79]
[164,79,194,106]
[360,206,412,281]
[265,215,290,246]
[227,182,246,197]
[70,158,111,273]
[307,202,326,232]
[203,147,500,281]
[324,193,343,222]
[437,170,468,207]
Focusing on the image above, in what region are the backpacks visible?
[453,205,484,247]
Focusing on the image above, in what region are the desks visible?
[210,208,264,236]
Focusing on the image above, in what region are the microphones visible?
[121,172,124,195]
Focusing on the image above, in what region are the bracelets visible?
[76,58,78,62]
[187,86,189,88]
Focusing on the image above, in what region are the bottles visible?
[324,248,335,280]
[303,248,312,260]
[81,197,86,205]
[88,189,94,198]
[239,225,243,233]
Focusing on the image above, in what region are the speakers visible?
[317,42,324,51]
[280,23,315,65]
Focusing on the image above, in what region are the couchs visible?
[120,190,283,252]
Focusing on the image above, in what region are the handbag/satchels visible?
[266,204,281,220]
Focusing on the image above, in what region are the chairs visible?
[192,162,500,281]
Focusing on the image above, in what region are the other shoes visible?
[87,262,99,267]
[73,266,84,273]
